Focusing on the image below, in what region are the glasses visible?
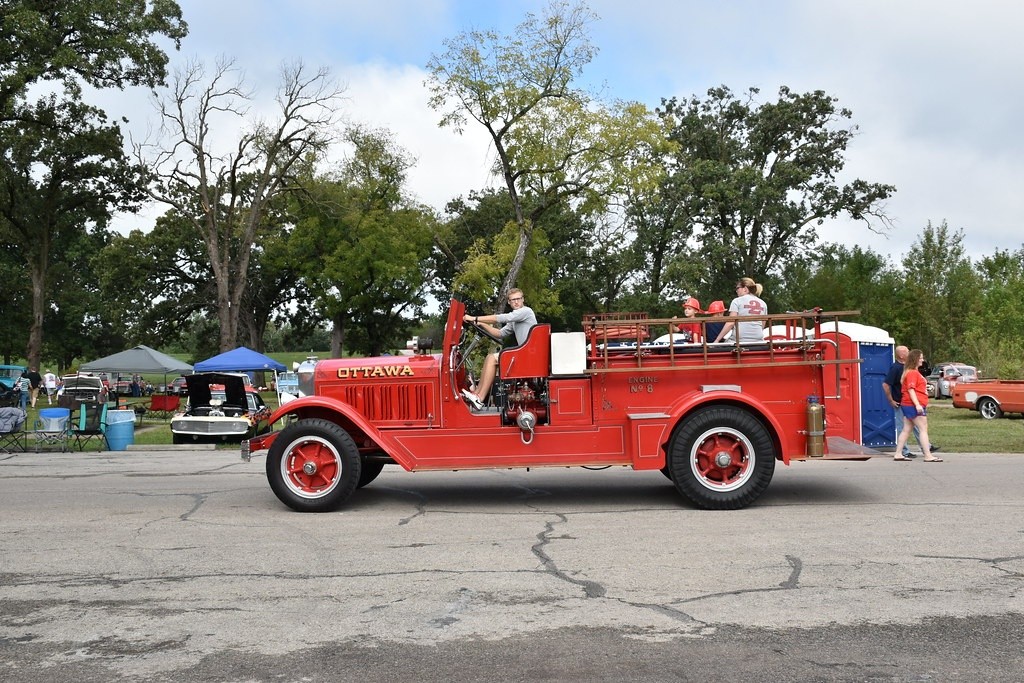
[508,296,524,303]
[736,287,746,290]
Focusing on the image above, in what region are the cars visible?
[112,375,146,395]
[924,362,978,400]
[270,372,299,397]
[56,376,108,409]
[166,377,189,395]
[0,365,28,398]
[170,371,273,444]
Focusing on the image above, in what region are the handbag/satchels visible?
[13,377,22,391]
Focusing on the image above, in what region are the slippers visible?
[924,456,943,462]
[894,456,912,461]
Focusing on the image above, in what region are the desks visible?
[108,402,135,410]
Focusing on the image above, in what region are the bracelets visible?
[476,316,478,324]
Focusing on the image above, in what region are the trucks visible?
[240,299,869,510]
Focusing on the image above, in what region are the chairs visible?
[499,323,550,380]
[0,396,180,455]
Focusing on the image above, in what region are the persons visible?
[15,373,33,410]
[713,278,767,343]
[706,301,728,343]
[27,366,42,411]
[42,369,56,405]
[133,374,140,397]
[894,349,944,462]
[672,298,704,344]
[882,346,940,458]
[461,288,537,410]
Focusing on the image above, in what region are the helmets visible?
[682,298,702,314]
[705,300,728,314]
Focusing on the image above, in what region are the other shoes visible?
[903,453,916,458]
[923,445,941,454]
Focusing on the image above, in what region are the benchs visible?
[651,345,770,353]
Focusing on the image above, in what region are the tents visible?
[73,345,194,424]
[186,347,287,426]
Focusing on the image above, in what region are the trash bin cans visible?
[105,409,135,451]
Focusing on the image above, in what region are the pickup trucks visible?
[952,380,1024,420]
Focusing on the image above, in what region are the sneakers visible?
[461,388,484,411]
